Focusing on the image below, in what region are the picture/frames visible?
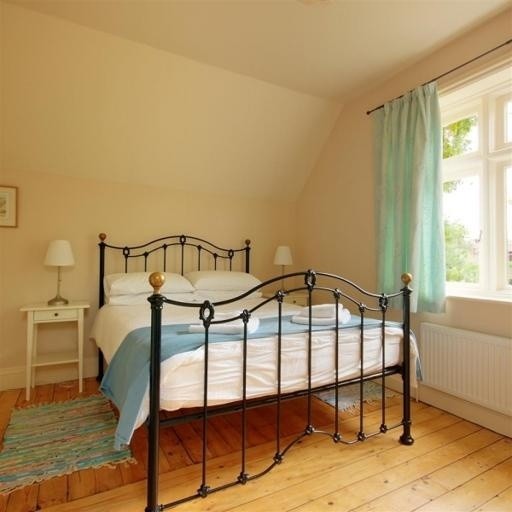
[0,185,20,228]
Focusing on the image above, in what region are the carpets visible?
[1,392,138,496]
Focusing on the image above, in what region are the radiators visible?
[417,322,511,418]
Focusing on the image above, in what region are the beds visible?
[95,231,415,511]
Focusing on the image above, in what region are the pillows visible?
[104,268,264,307]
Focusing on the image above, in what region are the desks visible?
[20,299,90,404]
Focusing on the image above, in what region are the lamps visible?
[272,245,294,295]
[44,239,76,306]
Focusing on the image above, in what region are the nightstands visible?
[262,292,309,306]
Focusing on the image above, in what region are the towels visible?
[291,302,351,327]
[187,310,258,335]
